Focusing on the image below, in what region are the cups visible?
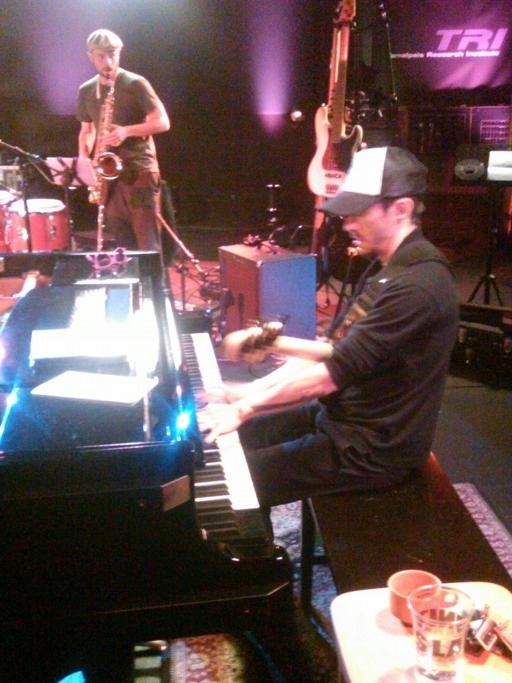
[386,567,442,627]
[407,584,476,683]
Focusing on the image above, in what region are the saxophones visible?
[88,68,123,206]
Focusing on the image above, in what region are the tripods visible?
[465,182,507,307]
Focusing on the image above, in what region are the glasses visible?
[85,247,129,269]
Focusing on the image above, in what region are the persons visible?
[72,28,172,253]
[194,144,461,541]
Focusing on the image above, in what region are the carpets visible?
[161,480,512,683]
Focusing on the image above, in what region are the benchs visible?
[285,451,510,617]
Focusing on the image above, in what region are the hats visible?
[86,29,124,50]
[316,146,429,215]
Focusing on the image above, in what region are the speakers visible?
[217,242,318,341]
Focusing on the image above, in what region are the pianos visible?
[0,258,295,649]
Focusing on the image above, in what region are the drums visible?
[1,198,72,254]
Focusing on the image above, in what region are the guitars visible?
[308,0,363,197]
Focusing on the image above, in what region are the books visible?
[26,325,126,370]
[28,368,160,406]
[44,156,98,187]
